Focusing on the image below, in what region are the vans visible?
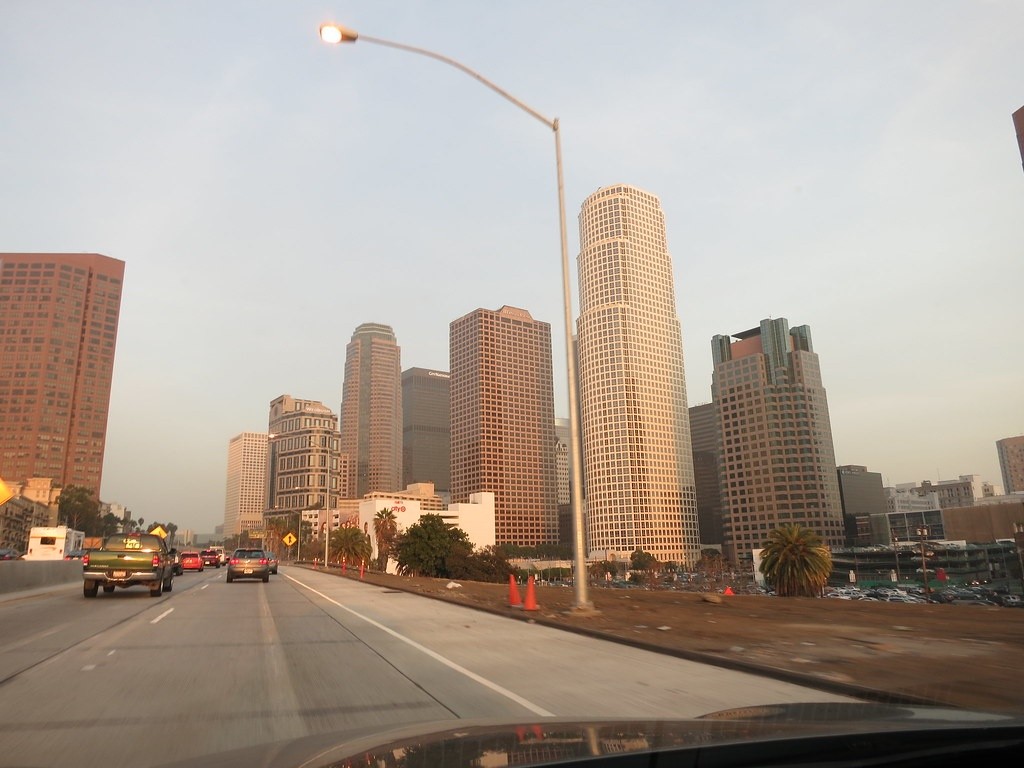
[208,545,228,567]
[27,525,86,561]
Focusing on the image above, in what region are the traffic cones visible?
[522,575,540,612]
[509,572,522,608]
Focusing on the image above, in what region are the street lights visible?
[268,433,331,568]
[322,23,592,611]
[275,505,301,560]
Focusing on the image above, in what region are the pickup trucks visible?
[82,532,177,598]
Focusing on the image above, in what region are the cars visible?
[225,550,232,563]
[179,551,204,572]
[169,552,183,576]
[226,547,272,583]
[199,549,222,568]
[250,550,279,575]
[0,549,28,561]
